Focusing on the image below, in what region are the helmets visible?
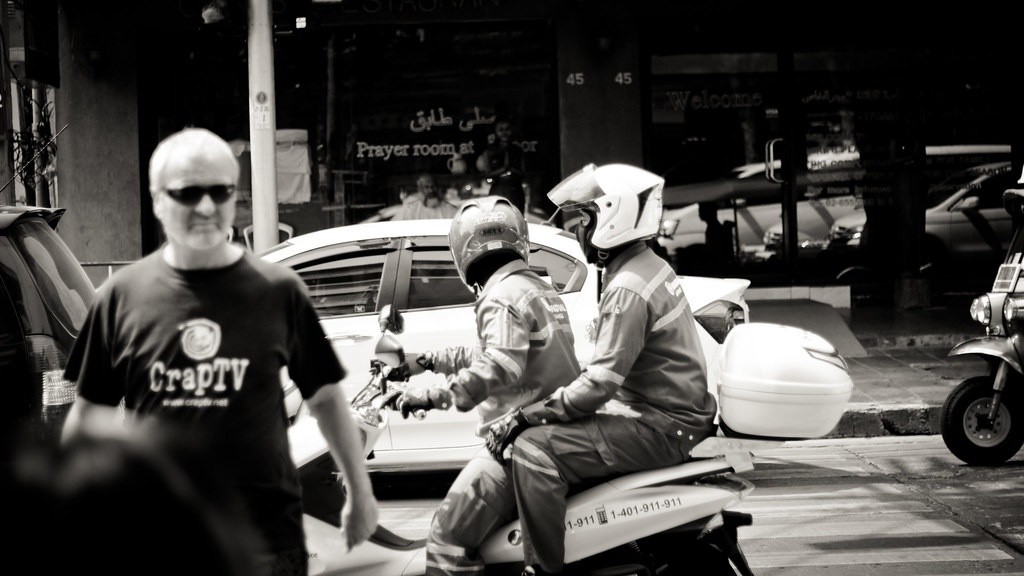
[447,195,529,296]
[572,163,666,266]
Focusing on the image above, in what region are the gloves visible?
[389,352,434,383]
[485,406,529,463]
[393,369,432,418]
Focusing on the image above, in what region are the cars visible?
[656,144,1015,272]
[234,217,750,482]
[831,161,1019,267]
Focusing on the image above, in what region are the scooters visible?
[938,241,1024,469]
[286,304,854,576]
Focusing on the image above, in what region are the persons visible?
[380,197,581,576]
[389,148,526,220]
[486,165,717,576]
[0,129,379,576]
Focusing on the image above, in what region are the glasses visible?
[161,184,238,207]
